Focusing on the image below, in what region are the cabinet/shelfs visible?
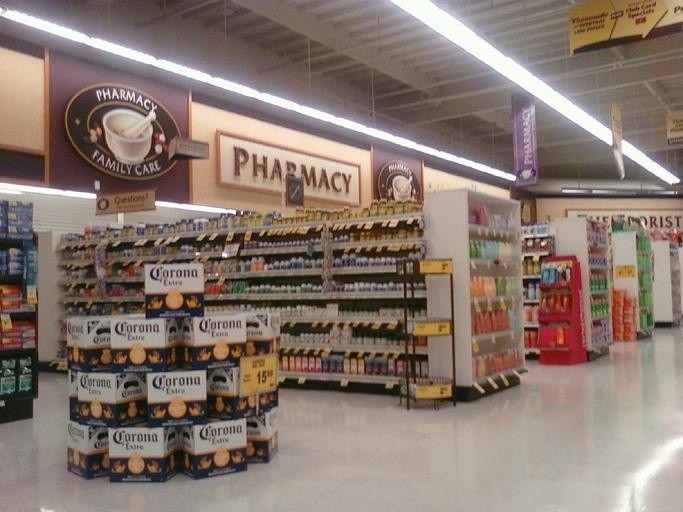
[0,230,39,424]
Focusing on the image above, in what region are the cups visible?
[392,176,413,201]
[99,108,152,165]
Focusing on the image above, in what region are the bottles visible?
[467,207,521,376]
[522,222,571,348]
[71,227,426,347]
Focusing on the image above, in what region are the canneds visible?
[273,199,421,242]
